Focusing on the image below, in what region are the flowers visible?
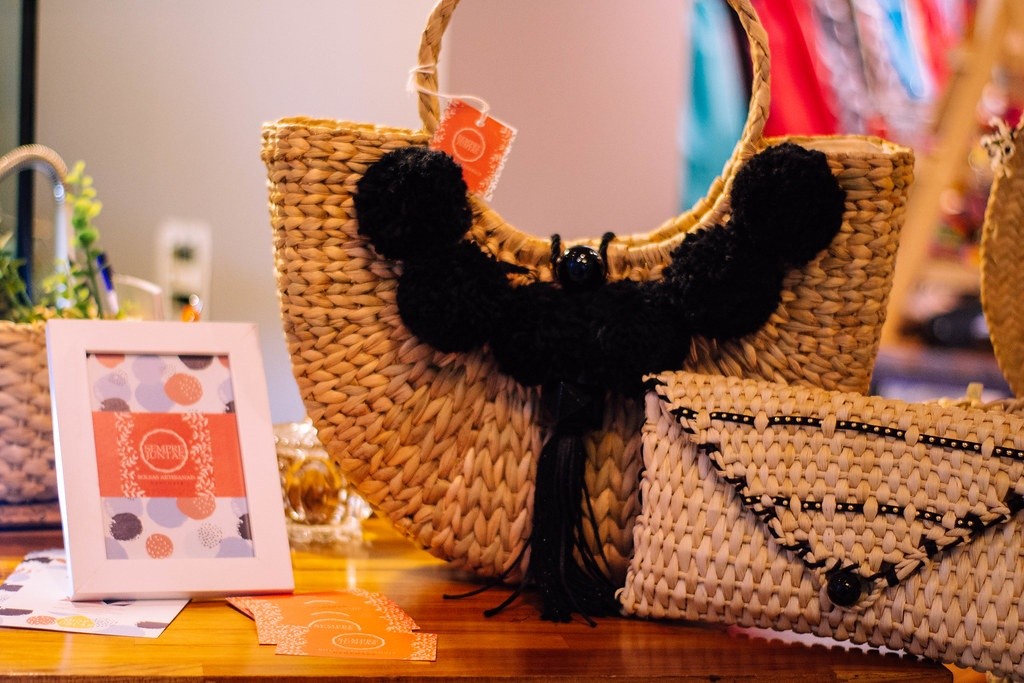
[0,162,202,325]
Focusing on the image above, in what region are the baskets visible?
[0,143,97,504]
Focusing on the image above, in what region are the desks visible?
[0,523,959,683]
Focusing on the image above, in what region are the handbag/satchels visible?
[262,0,914,626]
[616,368,1024,683]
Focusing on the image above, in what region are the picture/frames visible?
[44,316,295,601]
